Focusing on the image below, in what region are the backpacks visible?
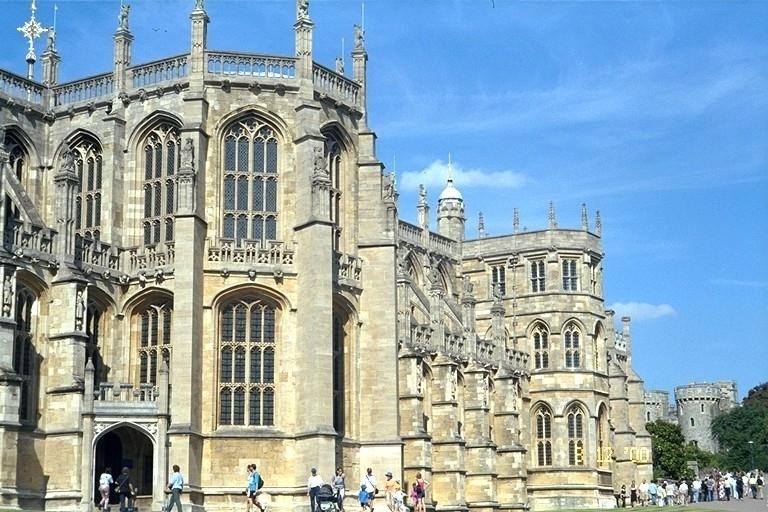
[253,472,264,489]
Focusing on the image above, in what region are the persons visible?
[357,468,431,512]
[307,469,324,512]
[115,467,134,511]
[621,468,763,507]
[248,464,267,512]
[332,467,345,512]
[245,465,252,511]
[163,465,184,511]
[98,467,115,512]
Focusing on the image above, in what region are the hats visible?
[385,472,392,477]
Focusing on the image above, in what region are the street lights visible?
[747,440,754,469]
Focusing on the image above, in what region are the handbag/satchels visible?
[98,484,108,492]
[416,484,422,495]
[375,489,378,494]
[114,487,120,492]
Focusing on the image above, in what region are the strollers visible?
[315,484,341,512]
